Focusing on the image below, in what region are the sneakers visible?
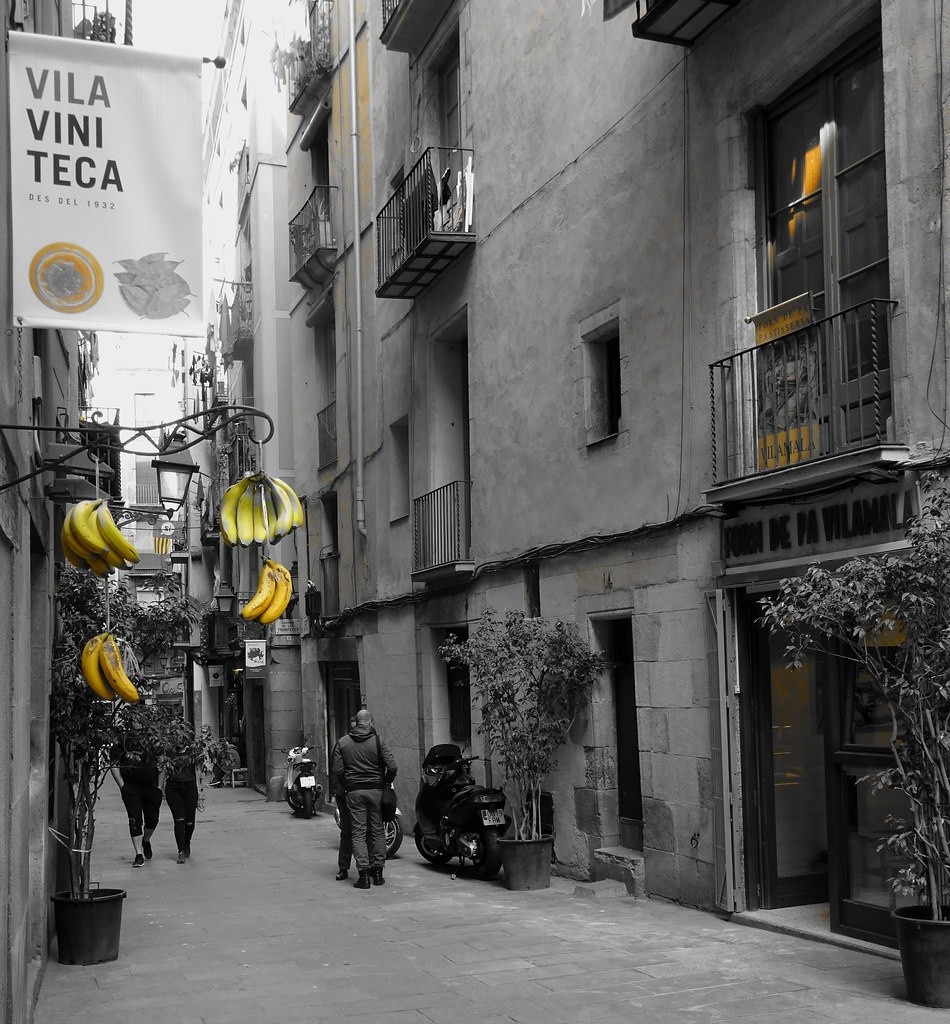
[132,854,145,867]
[142,836,152,859]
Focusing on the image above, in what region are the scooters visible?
[334,782,404,858]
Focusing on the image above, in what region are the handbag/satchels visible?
[381,783,397,822]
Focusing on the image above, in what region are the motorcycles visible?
[412,738,512,880]
[281,733,324,818]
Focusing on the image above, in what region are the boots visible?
[353,865,385,889]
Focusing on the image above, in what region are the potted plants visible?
[50,563,231,966]
[757,469,950,1008]
[436,607,609,890]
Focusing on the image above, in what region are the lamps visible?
[42,443,115,480]
[215,581,264,635]
[157,654,187,674]
[49,476,113,504]
[108,432,201,530]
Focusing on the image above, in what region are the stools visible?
[231,766,248,787]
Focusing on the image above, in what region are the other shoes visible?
[209,779,221,785]
[177,843,190,864]
[336,869,348,880]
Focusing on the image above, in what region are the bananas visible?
[82,631,140,702]
[239,555,292,624]
[219,470,303,548]
[60,499,140,578]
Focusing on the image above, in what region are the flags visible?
[5,30,208,339]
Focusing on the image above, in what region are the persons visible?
[329,710,397,889]
[209,738,248,789]
[110,719,167,867]
[167,746,207,863]
